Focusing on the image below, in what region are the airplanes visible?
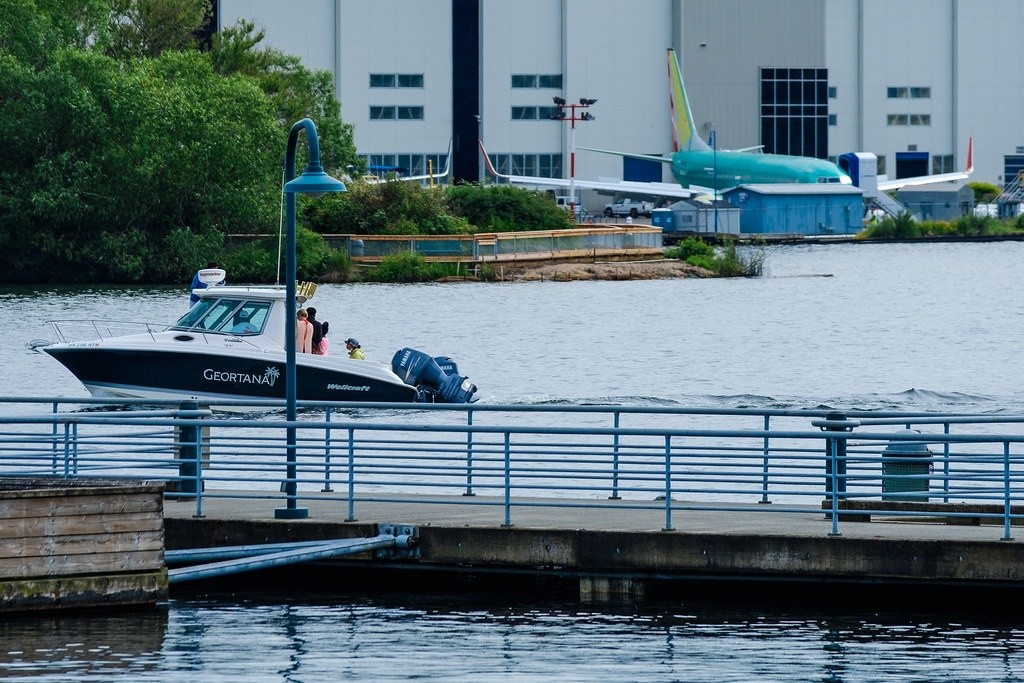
[477,46,974,228]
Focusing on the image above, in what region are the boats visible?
[32,168,480,405]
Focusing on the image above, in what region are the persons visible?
[343,337,365,360]
[189,262,226,329]
[295,307,330,355]
[230,309,259,334]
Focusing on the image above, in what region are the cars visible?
[604,198,654,219]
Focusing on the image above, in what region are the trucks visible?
[554,195,580,216]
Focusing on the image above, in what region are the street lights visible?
[272,118,347,519]
[549,95,597,221]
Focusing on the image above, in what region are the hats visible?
[321,322,329,337]
[344,338,359,347]
[306,307,316,317]
